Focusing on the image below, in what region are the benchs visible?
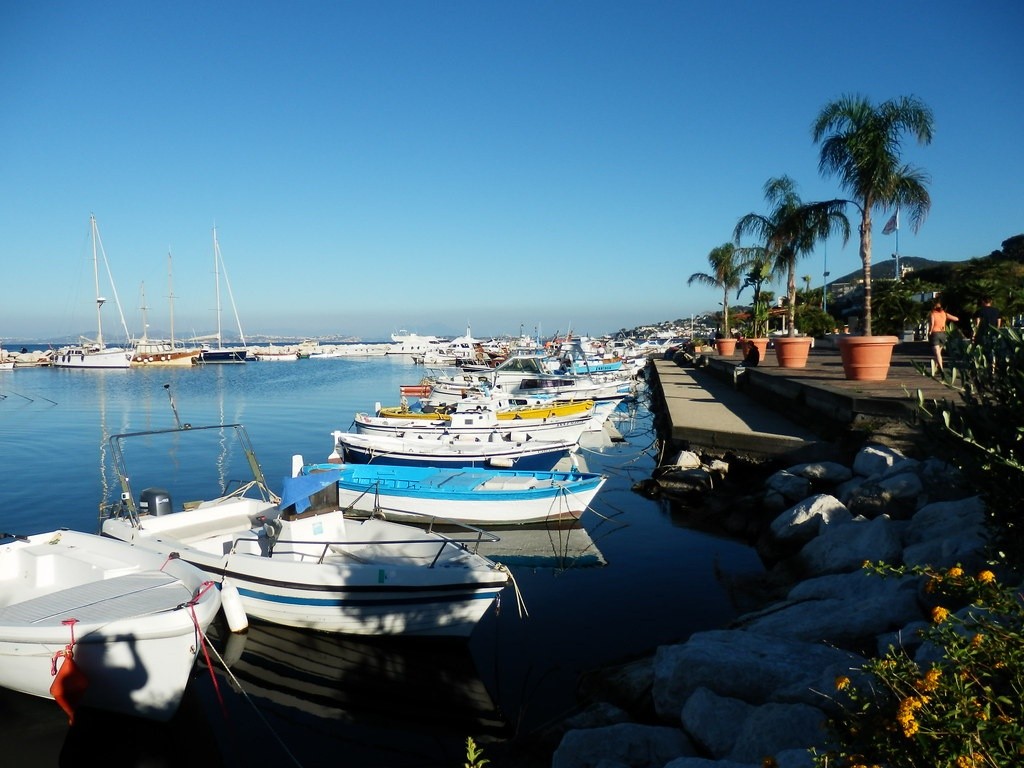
[21,543,137,585]
[152,513,257,546]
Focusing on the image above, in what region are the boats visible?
[430,520,611,568]
[104,423,512,642]
[0,524,222,726]
[195,608,510,747]
[660,337,690,350]
[252,323,663,470]
[552,399,639,473]
[291,451,607,526]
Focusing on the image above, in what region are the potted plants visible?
[687,241,767,359]
[731,174,865,366]
[727,244,783,363]
[809,92,939,380]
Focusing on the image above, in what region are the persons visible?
[728,340,759,386]
[559,359,573,385]
[928,295,1002,377]
[709,329,716,350]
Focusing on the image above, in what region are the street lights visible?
[719,302,724,333]
[893,254,901,279]
[823,271,830,316]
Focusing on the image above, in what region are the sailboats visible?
[196,221,249,364]
[46,211,139,369]
[132,247,204,366]
